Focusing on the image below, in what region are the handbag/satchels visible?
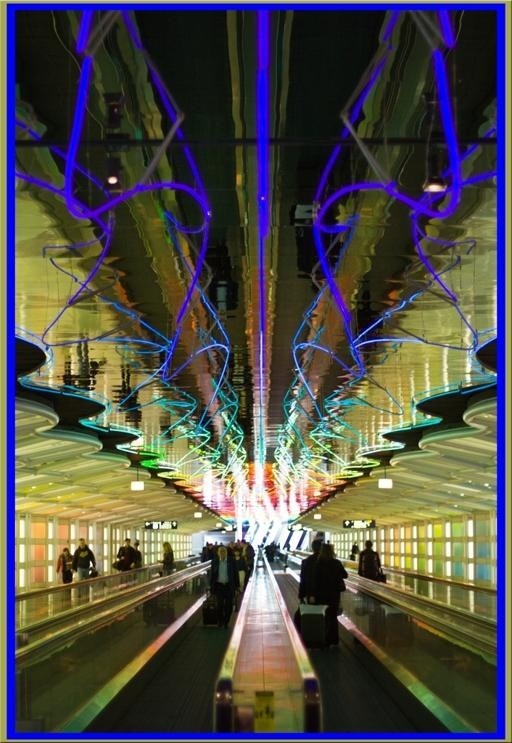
[112,560,123,570]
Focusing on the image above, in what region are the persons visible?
[156,542,176,576]
[56,547,75,584]
[133,540,143,567]
[72,538,96,581]
[116,538,137,571]
[307,544,348,647]
[298,540,322,604]
[264,541,281,562]
[359,540,381,614]
[197,540,256,629]
[351,541,358,554]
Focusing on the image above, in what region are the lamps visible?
[132,451,144,491]
[378,459,395,490]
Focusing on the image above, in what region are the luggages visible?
[203,589,220,626]
[143,598,176,623]
[299,602,328,650]
[370,602,414,647]
